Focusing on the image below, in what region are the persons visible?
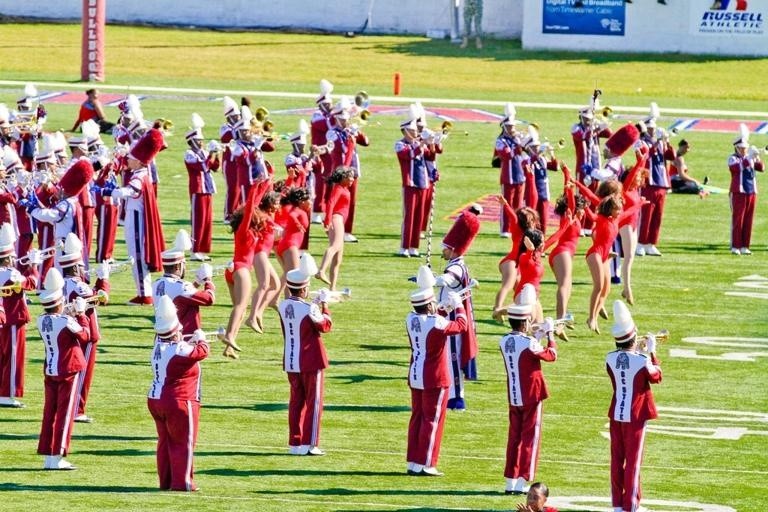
[499,317,557,496]
[605,333,662,512]
[728,138,765,257]
[218,139,355,360]
[61,263,108,423]
[0,249,39,408]
[152,249,215,343]
[278,287,332,455]
[184,88,370,263]
[460,0,483,49]
[429,247,478,411]
[393,103,443,259]
[405,292,467,476]
[37,297,93,470]
[516,482,559,512]
[147,328,209,492]
[491,87,706,342]
[1,86,173,306]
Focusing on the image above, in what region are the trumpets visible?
[757,145,767,155]
[307,288,350,304]
[436,278,480,311]
[1,240,134,309]
[2,118,40,136]
[314,140,335,156]
[182,325,225,344]
[532,314,575,330]
[419,120,453,138]
[346,91,371,125]
[149,118,174,132]
[187,261,235,279]
[665,127,681,141]
[543,139,567,150]
[215,139,236,153]
[636,329,670,344]
[251,107,275,139]
[595,106,614,119]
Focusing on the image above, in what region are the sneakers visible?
[50,455,80,470]
[646,243,662,256]
[407,461,416,474]
[410,462,444,476]
[635,243,646,256]
[514,476,532,495]
[741,247,752,254]
[504,477,518,495]
[0,397,27,408]
[74,414,94,423]
[731,247,741,255]
[622,503,649,512]
[613,505,624,512]
[396,247,409,258]
[43,455,52,470]
[288,445,302,455]
[298,445,326,456]
[409,246,420,257]
[445,397,466,412]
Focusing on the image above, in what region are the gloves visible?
[420,127,435,140]
[641,331,657,355]
[543,315,555,334]
[447,290,463,307]
[746,144,761,160]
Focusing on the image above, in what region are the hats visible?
[732,137,750,149]
[506,303,533,320]
[522,134,541,151]
[611,320,639,344]
[644,116,657,129]
[400,117,418,130]
[407,287,437,307]
[499,113,516,128]
[578,106,595,120]
[0,96,355,343]
[604,122,641,157]
[439,210,480,258]
[415,113,428,128]
